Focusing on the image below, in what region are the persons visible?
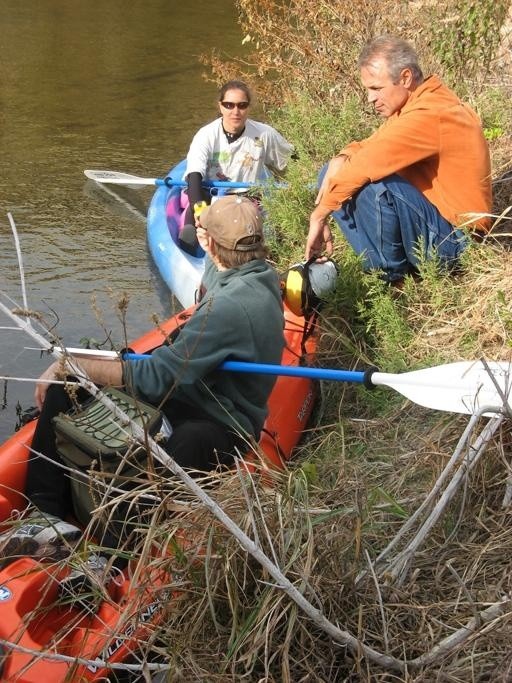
[0,194,284,614]
[306,34,492,295]
[177,82,297,257]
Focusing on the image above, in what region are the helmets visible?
[280,254,340,317]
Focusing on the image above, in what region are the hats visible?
[200,195,265,252]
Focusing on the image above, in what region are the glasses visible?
[221,101,249,109]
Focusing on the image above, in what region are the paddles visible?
[84,170,317,191]
[51,345,511,421]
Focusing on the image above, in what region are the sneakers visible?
[57,555,126,619]
[0,508,82,571]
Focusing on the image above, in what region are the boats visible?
[0,266,349,683]
[146,157,290,311]
